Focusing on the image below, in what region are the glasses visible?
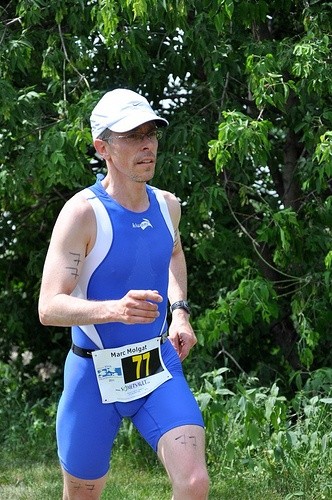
[102,126,157,143]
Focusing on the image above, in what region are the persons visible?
[38,88,210,499]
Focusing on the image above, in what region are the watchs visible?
[170,300,192,315]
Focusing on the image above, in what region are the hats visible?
[90,89,169,142]
